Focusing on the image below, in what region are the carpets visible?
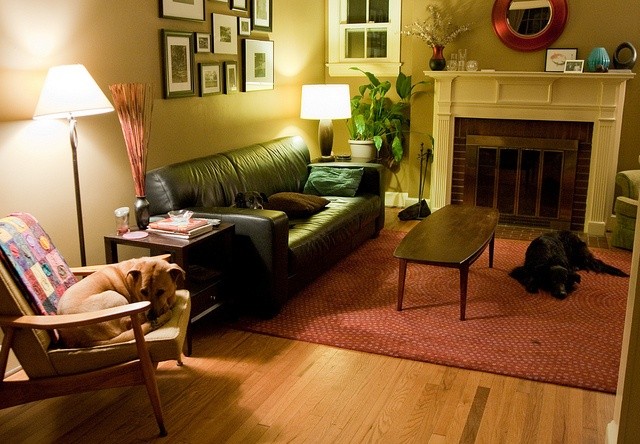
[238,204,633,393]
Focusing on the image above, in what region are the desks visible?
[103,218,236,355]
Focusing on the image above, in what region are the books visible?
[145,215,213,239]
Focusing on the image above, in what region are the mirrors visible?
[491,0,569,53]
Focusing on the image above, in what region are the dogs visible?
[229,190,296,229]
[57,257,186,348]
[509,231,630,300]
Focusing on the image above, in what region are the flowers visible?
[403,5,472,43]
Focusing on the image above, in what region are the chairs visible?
[0,212,190,438]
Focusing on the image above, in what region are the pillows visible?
[266,192,330,215]
[302,163,364,196]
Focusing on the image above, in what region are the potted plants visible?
[347,66,433,163]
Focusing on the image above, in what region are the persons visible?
[566,62,574,70]
[574,62,581,70]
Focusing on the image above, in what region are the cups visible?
[115,207,131,235]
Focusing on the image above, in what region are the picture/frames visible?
[160,0,206,23]
[239,17,251,34]
[252,0,273,31]
[162,30,195,99]
[195,32,212,53]
[241,37,274,92]
[230,0,247,11]
[544,47,577,72]
[198,62,223,97]
[564,60,583,73]
[225,61,237,95]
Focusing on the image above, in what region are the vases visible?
[134,196,150,230]
[428,42,445,71]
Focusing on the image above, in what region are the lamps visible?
[32,64,116,280]
[300,84,351,161]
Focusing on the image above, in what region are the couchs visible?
[614,154,640,250]
[140,136,385,315]
